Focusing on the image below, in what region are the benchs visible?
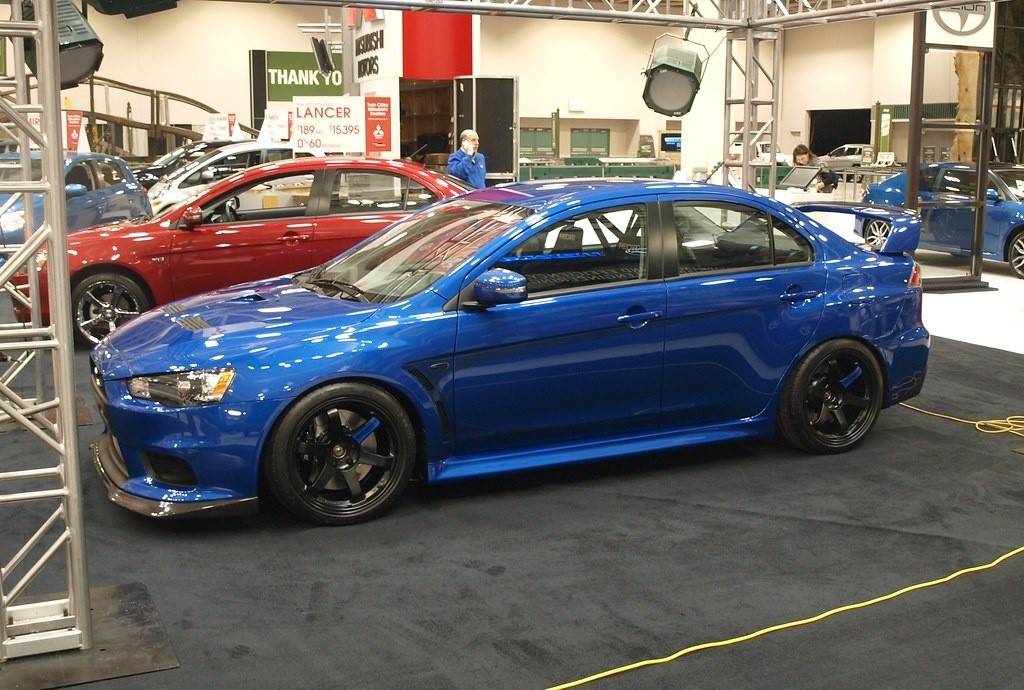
[679,238,808,266]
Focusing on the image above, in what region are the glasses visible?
[796,157,804,162]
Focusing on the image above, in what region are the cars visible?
[855,160,1024,278]
[817,143,870,182]
[132,140,247,192]
[0,154,154,276]
[10,156,531,352]
[144,141,350,218]
[89,178,921,522]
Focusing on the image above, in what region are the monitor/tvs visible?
[780,166,822,187]
[310,37,336,78]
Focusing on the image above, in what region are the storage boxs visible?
[425,153,451,170]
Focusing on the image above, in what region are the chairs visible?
[607,233,642,268]
[65,165,88,195]
[540,226,584,275]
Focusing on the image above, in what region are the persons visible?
[793,144,838,193]
[447,129,486,188]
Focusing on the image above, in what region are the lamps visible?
[641,32,710,117]
[8,1,105,90]
